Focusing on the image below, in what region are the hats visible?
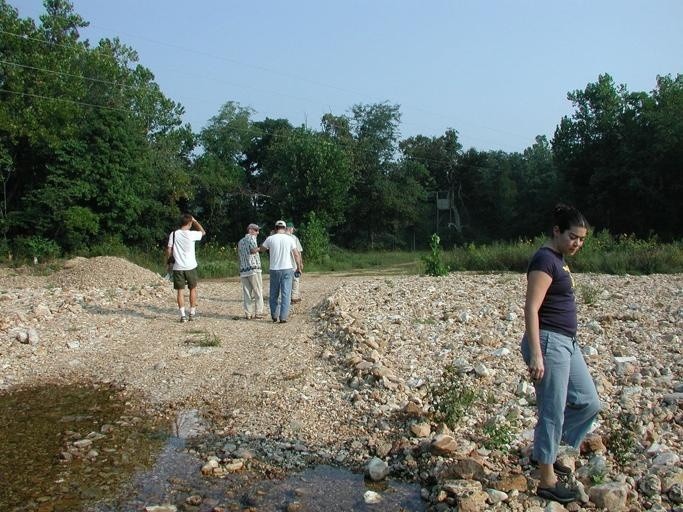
[275,220,293,229]
[247,224,261,230]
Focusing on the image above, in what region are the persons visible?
[283,222,302,304]
[236,222,263,321]
[164,212,208,324]
[518,202,601,504]
[258,219,302,323]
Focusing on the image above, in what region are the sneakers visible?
[529,458,570,476]
[536,482,575,504]
